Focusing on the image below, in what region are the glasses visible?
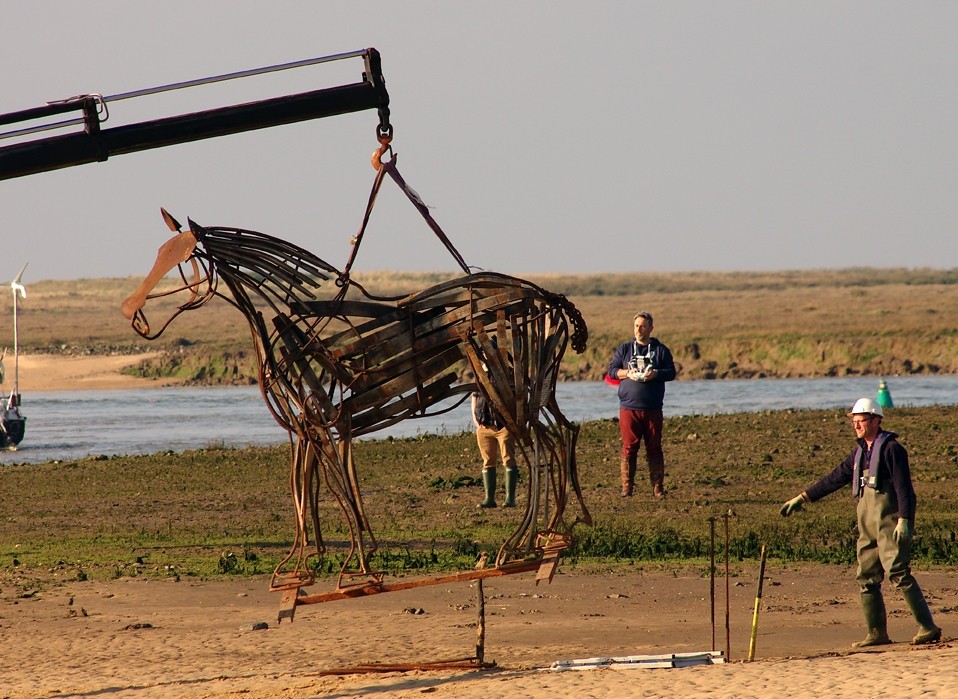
[850,416,875,427]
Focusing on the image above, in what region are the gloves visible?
[893,517,910,547]
[779,494,807,517]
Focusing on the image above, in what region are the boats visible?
[0,262,36,450]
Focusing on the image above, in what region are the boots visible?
[851,594,890,647]
[648,459,666,501]
[501,466,518,507]
[477,466,498,508]
[901,588,942,645]
[620,455,638,496]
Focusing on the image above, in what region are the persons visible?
[607,311,676,499]
[471,339,519,508]
[780,397,941,648]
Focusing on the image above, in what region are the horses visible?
[120,207,593,593]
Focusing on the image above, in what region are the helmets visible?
[846,398,884,419]
